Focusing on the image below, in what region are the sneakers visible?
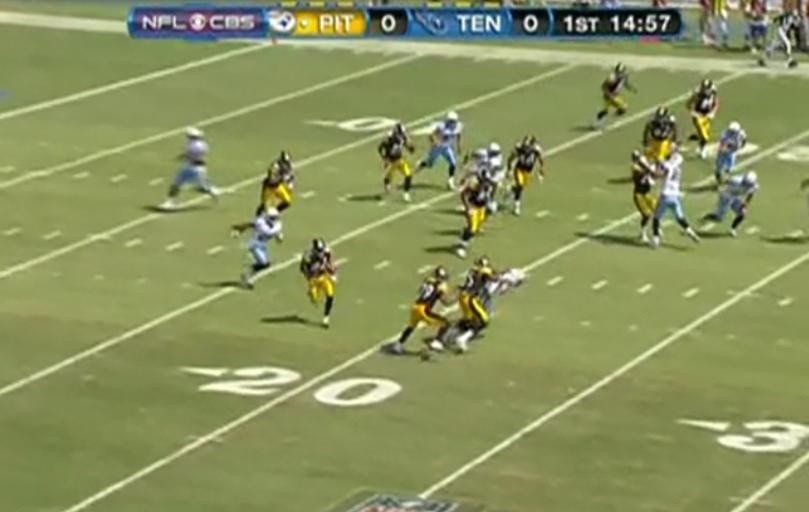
[638,214,738,246]
[404,192,412,203]
[323,316,331,325]
[392,327,469,354]
[242,273,253,288]
[488,183,521,216]
[455,243,468,259]
[447,183,455,190]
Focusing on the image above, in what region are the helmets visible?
[446,111,457,121]
[744,172,757,184]
[479,257,489,266]
[728,122,740,131]
[313,237,325,251]
[394,123,405,133]
[472,133,536,163]
[616,63,627,75]
[435,267,447,278]
[702,79,712,89]
[657,106,669,116]
[267,150,290,222]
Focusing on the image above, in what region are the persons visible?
[397,110,463,190]
[506,133,543,216]
[628,76,762,248]
[157,126,222,211]
[483,142,504,221]
[294,1,809,69]
[391,266,460,355]
[253,150,297,220]
[300,238,337,328]
[448,268,524,342]
[379,122,415,202]
[230,206,283,291]
[587,63,638,132]
[447,254,509,353]
[451,148,502,259]
